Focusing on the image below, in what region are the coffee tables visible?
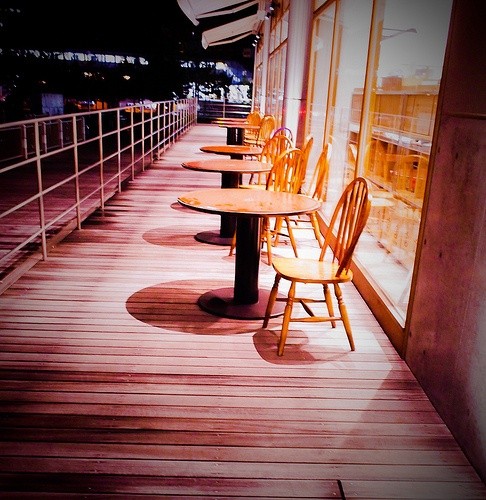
[219,124,259,146]
[211,121,244,125]
[345,132,422,261]
[181,159,272,188]
[216,117,247,121]
[200,145,263,160]
[179,187,322,319]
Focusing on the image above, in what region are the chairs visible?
[228,111,335,259]
[263,177,371,358]
